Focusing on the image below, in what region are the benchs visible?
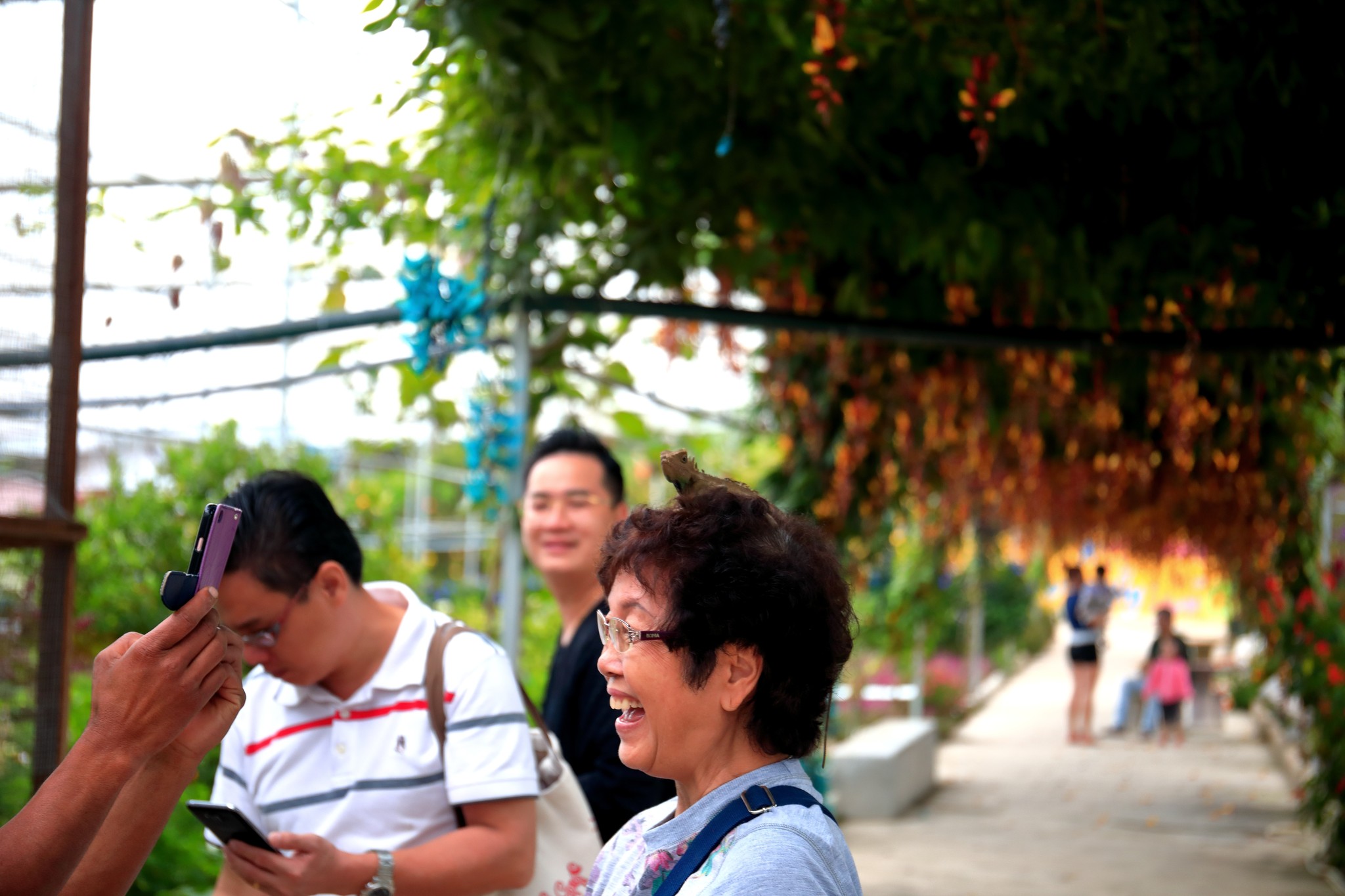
[825,716,936,817]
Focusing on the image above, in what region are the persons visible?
[518,427,677,845]
[202,469,539,896]
[586,486,865,896]
[1062,561,1195,746]
[0,583,246,896]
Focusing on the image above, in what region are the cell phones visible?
[187,800,283,856]
[172,503,242,611]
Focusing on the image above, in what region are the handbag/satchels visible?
[425,624,604,896]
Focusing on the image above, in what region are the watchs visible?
[358,849,395,896]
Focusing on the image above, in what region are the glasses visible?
[597,610,685,654]
[236,570,316,651]
